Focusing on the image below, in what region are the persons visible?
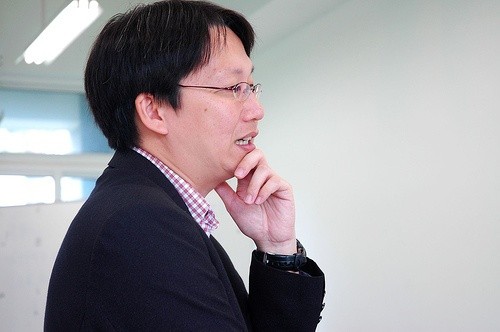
[43,0,325,332]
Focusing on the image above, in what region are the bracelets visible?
[254,238,307,271]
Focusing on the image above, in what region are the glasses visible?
[178,82,262,102]
